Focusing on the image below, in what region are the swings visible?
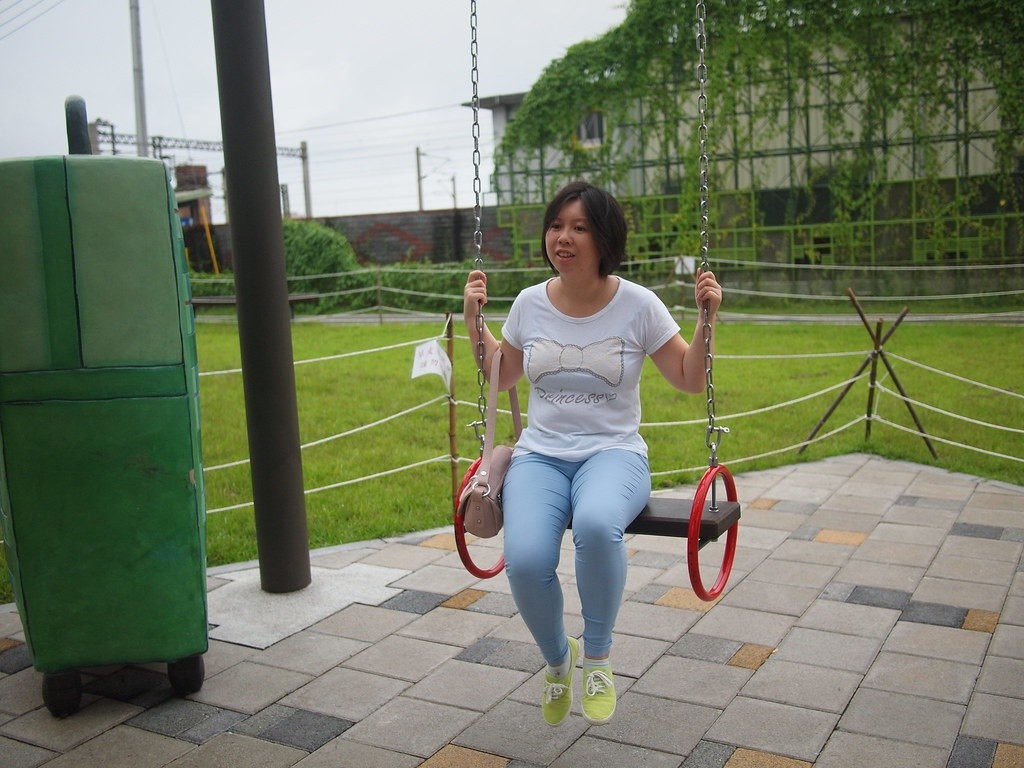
[451,0,746,599]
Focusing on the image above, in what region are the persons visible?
[462,182,725,730]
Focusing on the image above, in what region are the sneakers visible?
[542,636,579,727]
[582,659,616,725]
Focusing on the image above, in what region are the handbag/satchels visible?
[457,348,523,538]
